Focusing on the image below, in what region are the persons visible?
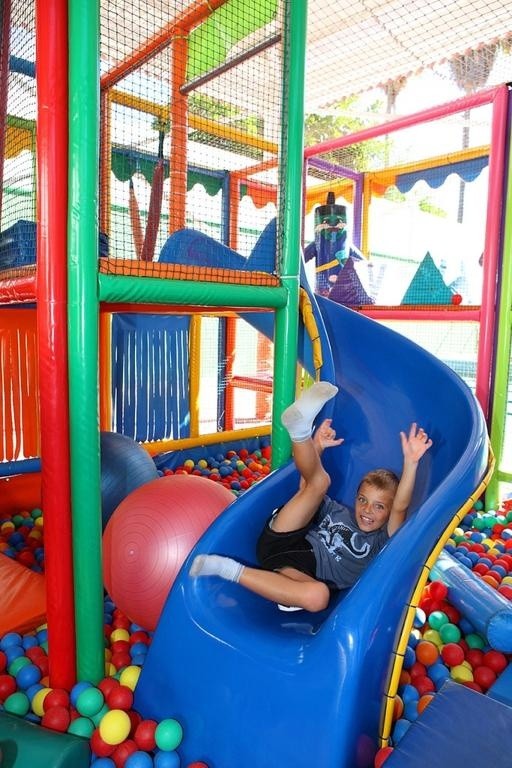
[183,378,436,618]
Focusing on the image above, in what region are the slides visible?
[133,218,493,766]
